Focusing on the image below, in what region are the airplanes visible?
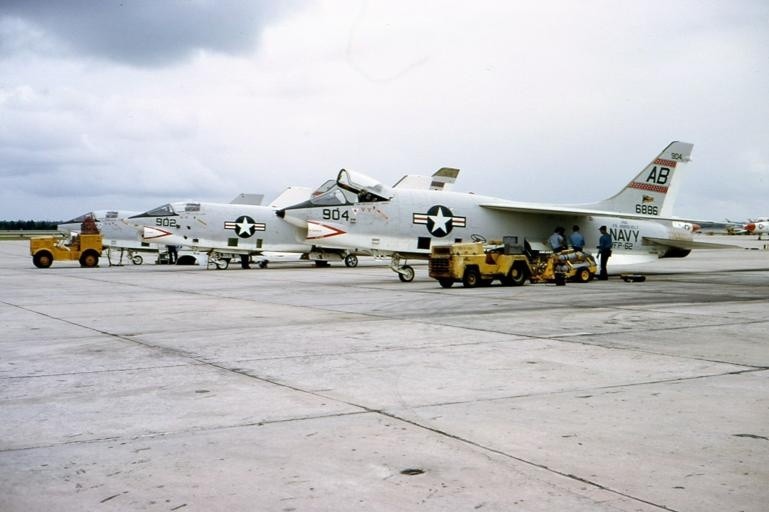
[725,214,769,241]
[274,133,765,287]
[55,189,270,271]
[120,165,464,272]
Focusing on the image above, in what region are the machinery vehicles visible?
[29,223,106,272]
[426,227,539,291]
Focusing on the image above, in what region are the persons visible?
[258,259,269,269]
[165,245,178,264]
[548,225,566,254]
[596,225,612,281]
[240,255,251,269]
[568,224,585,252]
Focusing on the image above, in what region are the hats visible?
[598,226,606,230]
